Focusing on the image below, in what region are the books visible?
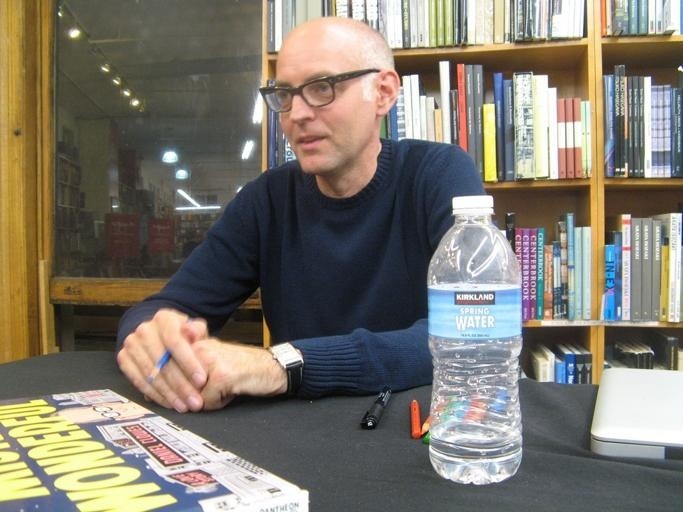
[266,60,683,183]
[267,1,682,51]
[0,388,309,512]
[526,329,683,383]
[506,212,682,324]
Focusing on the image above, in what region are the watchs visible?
[270,341,304,396]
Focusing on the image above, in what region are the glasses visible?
[259,67,381,113]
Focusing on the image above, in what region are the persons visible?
[116,17,486,413]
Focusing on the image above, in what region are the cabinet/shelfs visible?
[260,0,683,385]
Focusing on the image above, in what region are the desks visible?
[0,351,683,511]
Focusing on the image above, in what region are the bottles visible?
[427,196,522,485]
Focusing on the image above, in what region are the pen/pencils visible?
[146,314,193,385]
[361,384,391,430]
[410,399,430,443]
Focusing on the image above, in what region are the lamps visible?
[56,0,145,114]
